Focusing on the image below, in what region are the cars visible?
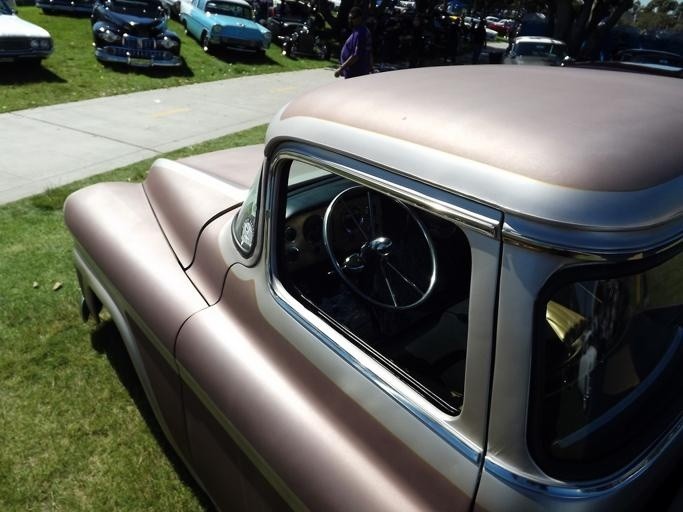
[181,0,271,56]
[91,0,182,69]
[63,62,683,512]
[162,0,181,17]
[0,0,55,63]
[504,27,683,80]
[36,0,93,17]
[375,1,513,46]
[265,0,332,60]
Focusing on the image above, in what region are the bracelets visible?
[339,65,345,70]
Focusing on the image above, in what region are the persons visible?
[471,22,487,64]
[520,2,554,57]
[443,12,451,26]
[446,18,460,62]
[409,14,424,67]
[335,7,373,77]
